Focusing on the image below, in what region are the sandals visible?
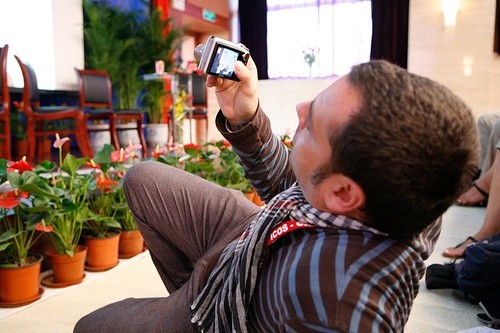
[443,236,479,257]
[455,183,489,207]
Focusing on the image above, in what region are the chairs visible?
[0,43,13,159]
[166,69,208,146]
[14,54,96,162]
[74,65,148,159]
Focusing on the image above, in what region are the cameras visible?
[194,35,249,82]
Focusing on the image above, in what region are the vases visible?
[0,253,45,308]
[120,229,144,256]
[245,190,254,202]
[46,244,89,282]
[253,188,265,206]
[85,229,123,272]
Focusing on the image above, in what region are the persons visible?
[443,112,500,258]
[74,56,481,333]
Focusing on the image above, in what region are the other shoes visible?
[426,256,464,290]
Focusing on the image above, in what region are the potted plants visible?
[82,0,193,160]
[0,131,293,266]
